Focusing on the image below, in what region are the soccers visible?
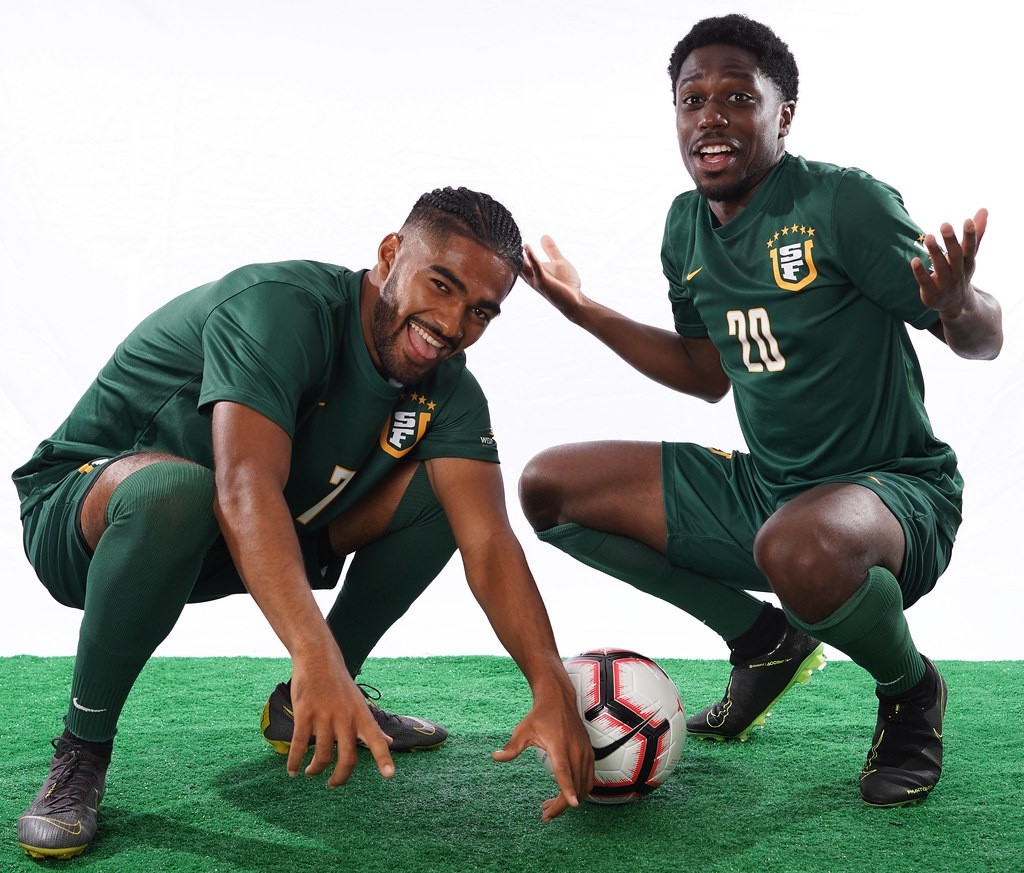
[537,648,687,804]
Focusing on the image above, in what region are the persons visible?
[9,185,595,861]
[517,12,1004,807]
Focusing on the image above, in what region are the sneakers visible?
[263,682,451,756]
[683,621,824,741]
[17,744,116,858]
[858,653,949,808]
